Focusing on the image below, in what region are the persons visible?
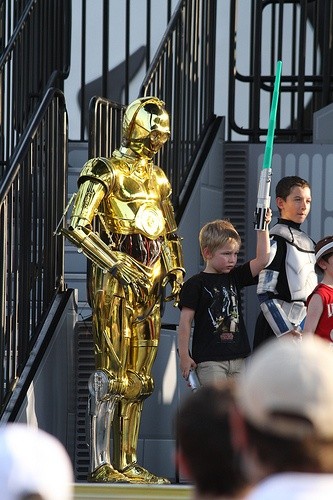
[176,207,272,391]
[59,95,187,483]
[0,422,73,500]
[254,176,333,355]
[173,334,333,500]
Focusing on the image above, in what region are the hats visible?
[0,423,74,500]
[235,334,332,440]
[314,235,333,275]
